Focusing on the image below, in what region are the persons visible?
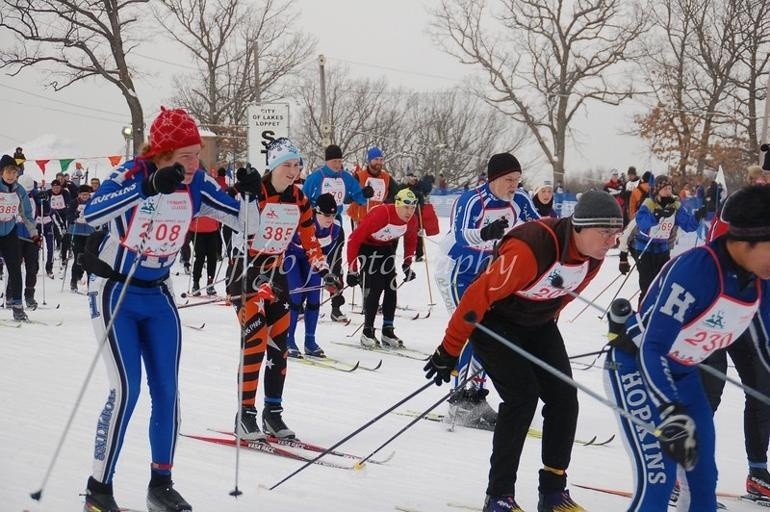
[225,143,348,444]
[422,189,625,512]
[601,179,768,512]
[435,151,541,429]
[80,104,260,512]
[689,210,770,512]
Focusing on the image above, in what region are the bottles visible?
[609,298,632,324]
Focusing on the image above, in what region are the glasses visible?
[592,229,624,239]
[394,197,418,205]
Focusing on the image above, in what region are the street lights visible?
[122,125,133,162]
[433,143,447,190]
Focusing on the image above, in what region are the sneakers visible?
[330,308,347,322]
[361,325,403,349]
[145,479,193,512]
[443,390,500,432]
[191,286,217,296]
[47,271,54,279]
[5,296,38,321]
[183,263,192,275]
[287,341,324,358]
[82,476,119,511]
[481,493,523,512]
[745,469,770,497]
[234,404,296,442]
[537,468,588,512]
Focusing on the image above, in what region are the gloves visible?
[346,271,361,286]
[233,162,262,202]
[424,344,460,386]
[142,161,185,197]
[319,269,343,291]
[657,403,700,472]
[362,186,374,198]
[401,264,415,282]
[618,251,631,275]
[255,276,278,305]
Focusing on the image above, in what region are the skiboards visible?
[392,407,615,445]
[569,358,596,370]
[178,428,395,470]
[318,303,432,326]
[1,304,63,328]
[182,322,206,330]
[287,355,383,372]
[332,341,433,361]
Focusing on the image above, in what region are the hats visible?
[572,189,624,228]
[533,177,554,196]
[0,154,16,168]
[487,153,522,183]
[730,185,769,242]
[324,145,344,161]
[366,146,383,161]
[314,193,337,214]
[79,185,90,192]
[265,136,304,172]
[149,106,203,155]
[394,188,418,208]
[51,179,60,186]
[610,166,673,195]
